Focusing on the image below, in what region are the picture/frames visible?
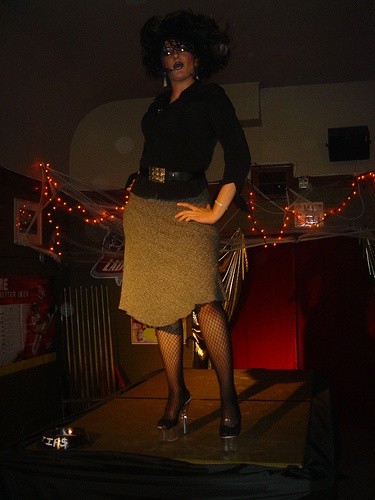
[13,196,43,246]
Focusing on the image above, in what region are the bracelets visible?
[215,199,230,211]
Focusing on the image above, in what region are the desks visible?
[208,229,375,371]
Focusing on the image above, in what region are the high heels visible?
[157,392,192,431]
[219,403,241,439]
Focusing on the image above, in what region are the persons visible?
[115,10,250,438]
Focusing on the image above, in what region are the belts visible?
[139,166,209,185]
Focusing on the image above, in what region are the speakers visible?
[328,126,370,162]
